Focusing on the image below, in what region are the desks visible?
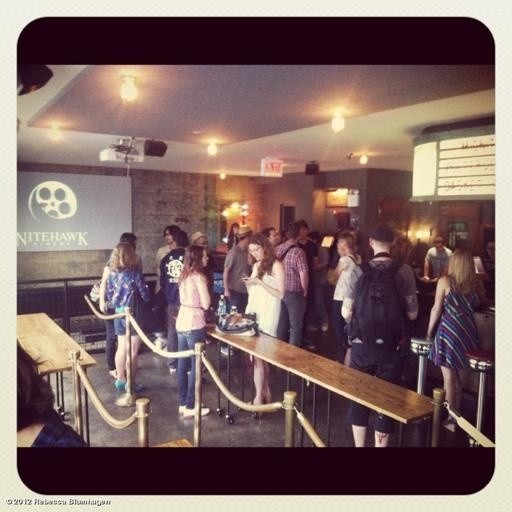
[17,312,97,447]
[206,316,437,448]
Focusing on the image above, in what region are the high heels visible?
[114,380,144,393]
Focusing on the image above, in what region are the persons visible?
[423,236,453,282]
[242,232,285,406]
[308,231,330,333]
[274,221,309,348]
[153,224,181,337]
[263,227,279,246]
[330,227,358,269]
[227,222,240,250]
[98,232,143,378]
[159,230,193,375]
[190,231,214,345]
[340,224,419,448]
[426,248,488,433]
[103,242,151,392]
[175,245,212,419]
[219,225,254,356]
[16,339,89,447]
[325,234,364,362]
[296,218,319,333]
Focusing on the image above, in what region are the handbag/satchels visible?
[347,264,408,349]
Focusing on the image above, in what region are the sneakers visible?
[177,405,187,413]
[182,408,211,418]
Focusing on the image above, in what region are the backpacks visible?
[119,280,155,332]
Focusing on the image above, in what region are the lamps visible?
[347,150,367,165]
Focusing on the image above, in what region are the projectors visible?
[97,145,146,165]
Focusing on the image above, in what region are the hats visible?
[235,223,255,238]
[189,230,209,246]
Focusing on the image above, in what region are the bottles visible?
[218,294,227,315]
[230,305,238,313]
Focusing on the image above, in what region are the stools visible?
[411,334,436,394]
[463,347,494,447]
